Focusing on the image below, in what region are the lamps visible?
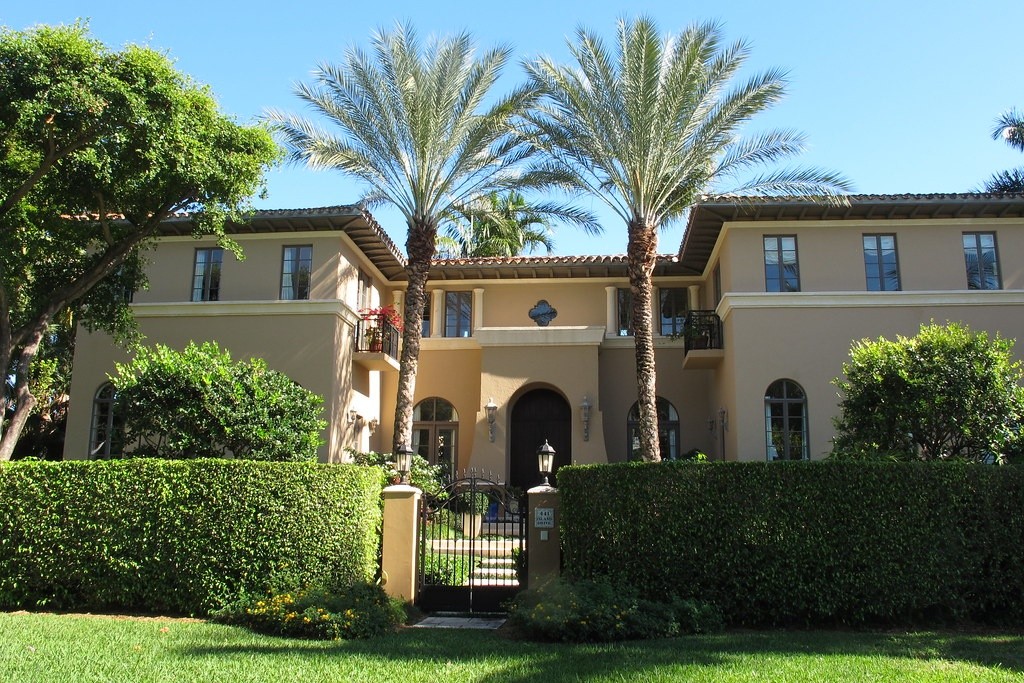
[536,438,555,486]
[707,416,718,442]
[580,395,592,422]
[717,407,729,432]
[395,439,413,486]
[484,399,497,424]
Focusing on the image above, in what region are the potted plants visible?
[665,311,710,349]
[454,491,490,539]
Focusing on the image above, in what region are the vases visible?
[369,339,390,354]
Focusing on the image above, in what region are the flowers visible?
[358,304,403,339]
